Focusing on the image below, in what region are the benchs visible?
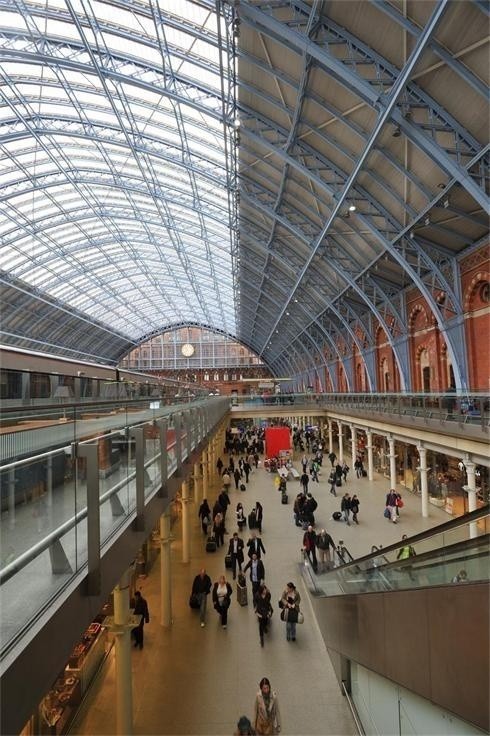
[277,465,299,480]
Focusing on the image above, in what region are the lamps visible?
[318,196,451,319]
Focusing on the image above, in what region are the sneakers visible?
[200,623,205,627]
[222,624,226,629]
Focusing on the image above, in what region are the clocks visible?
[182,344,194,356]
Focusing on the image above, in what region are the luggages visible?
[333,511,343,520]
[240,484,246,491]
[236,582,248,606]
[189,596,199,608]
[248,511,255,529]
[336,480,341,486]
[282,495,288,504]
[242,517,246,526]
[206,543,215,551]
[225,554,232,568]
[362,470,367,476]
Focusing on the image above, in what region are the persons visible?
[198,487,265,548]
[261,388,296,406]
[123,379,176,397]
[215,421,266,493]
[129,592,150,650]
[291,424,327,454]
[277,451,365,497]
[253,677,281,736]
[292,490,360,570]
[451,570,468,583]
[234,716,256,736]
[384,488,417,580]
[445,383,456,413]
[190,531,301,648]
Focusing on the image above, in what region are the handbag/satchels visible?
[238,574,246,586]
[398,500,403,507]
[280,608,304,624]
[384,509,390,518]
[278,600,284,607]
[328,478,332,483]
